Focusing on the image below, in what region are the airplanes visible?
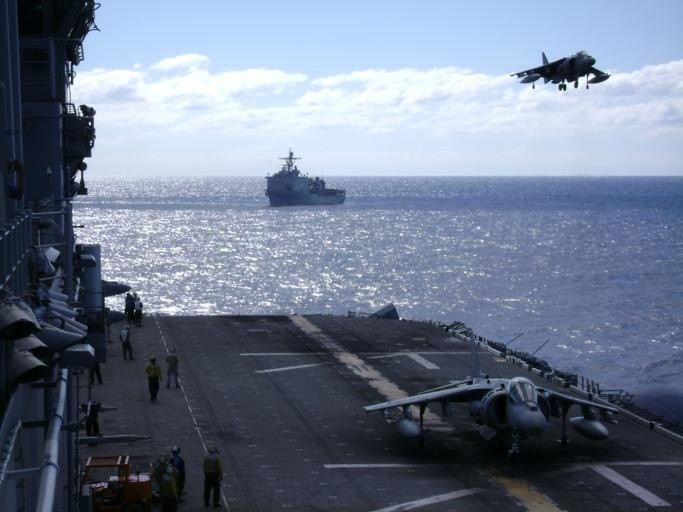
[509,49,612,91]
[362,329,620,463]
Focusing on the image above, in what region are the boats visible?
[265,146,346,206]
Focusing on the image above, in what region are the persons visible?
[169,445,186,504]
[81,401,100,446]
[144,355,162,401]
[89,362,103,385]
[150,456,179,511]
[120,325,135,361]
[165,347,179,388]
[134,297,143,327]
[124,295,135,323]
[125,293,133,321]
[203,446,224,508]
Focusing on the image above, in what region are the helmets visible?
[169,347,177,353]
[171,445,181,455]
[124,325,129,329]
[150,356,156,362]
[206,445,216,452]
